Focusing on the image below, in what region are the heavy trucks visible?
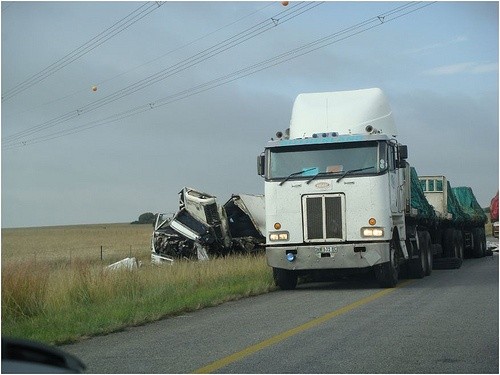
[255,88,489,292]
